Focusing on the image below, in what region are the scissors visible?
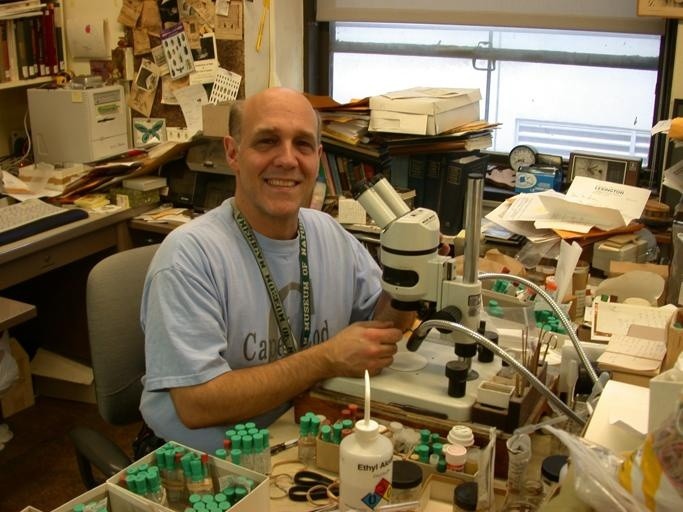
[540,329,558,366]
[291,469,339,501]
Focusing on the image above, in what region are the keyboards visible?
[0,199,70,234]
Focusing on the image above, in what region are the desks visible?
[269,268,608,512]
[0,154,162,339]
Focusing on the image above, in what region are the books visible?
[583,300,674,376]
[315,87,503,198]
[0,1,64,83]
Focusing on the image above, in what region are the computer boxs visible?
[27,83,128,170]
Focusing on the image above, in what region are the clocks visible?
[508,143,538,173]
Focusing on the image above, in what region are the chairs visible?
[70,241,160,487]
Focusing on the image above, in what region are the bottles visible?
[584,288,618,308]
[119,422,272,512]
[73,501,109,512]
[299,369,478,511]
[541,456,568,494]
[488,267,570,335]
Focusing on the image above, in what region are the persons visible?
[133,87,451,467]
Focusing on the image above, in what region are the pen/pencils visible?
[270,439,298,455]
